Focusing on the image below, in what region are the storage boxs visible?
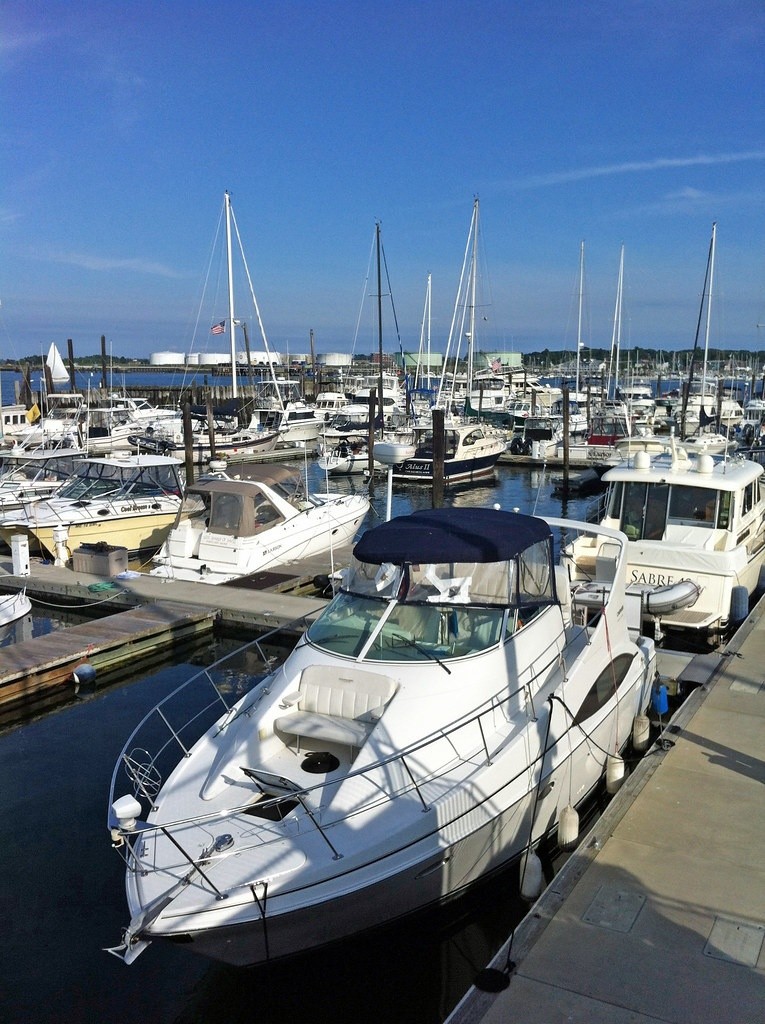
[73,546,129,576]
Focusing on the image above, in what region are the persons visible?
[351,440,358,451]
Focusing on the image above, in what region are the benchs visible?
[662,524,732,551]
[275,664,396,764]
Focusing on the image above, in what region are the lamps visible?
[112,793,141,829]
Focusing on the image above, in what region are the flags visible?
[211,320,225,335]
[26,404,40,423]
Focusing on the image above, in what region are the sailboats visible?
[40,340,70,383]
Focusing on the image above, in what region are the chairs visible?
[454,611,505,645]
[400,606,440,644]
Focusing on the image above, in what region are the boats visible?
[0,188,765,646]
[101,506,668,968]
